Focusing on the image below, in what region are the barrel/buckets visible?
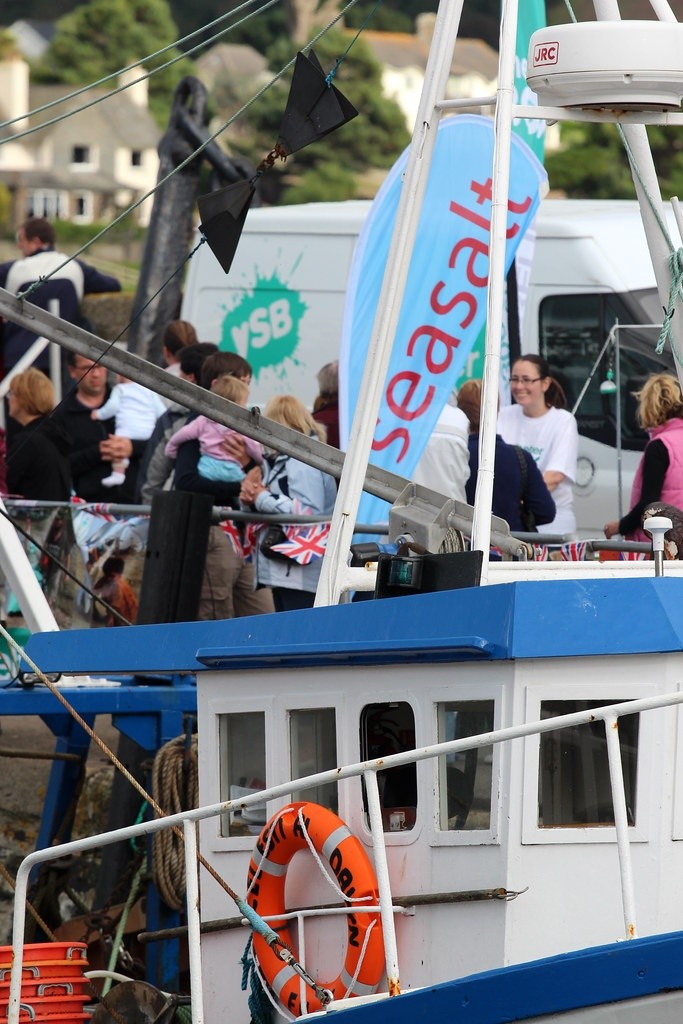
[1,942,93,1024]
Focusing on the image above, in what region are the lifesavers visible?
[244,798,388,1017]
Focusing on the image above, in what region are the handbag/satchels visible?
[513,444,539,533]
[259,519,312,566]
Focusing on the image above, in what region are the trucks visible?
[179,200,683,561]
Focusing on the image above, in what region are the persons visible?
[0,218,683,630]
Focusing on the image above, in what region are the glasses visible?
[509,377,545,384]
[73,365,102,372]
[6,392,16,398]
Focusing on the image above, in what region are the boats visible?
[0,0,683,1024]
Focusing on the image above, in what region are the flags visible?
[268,494,337,566]
